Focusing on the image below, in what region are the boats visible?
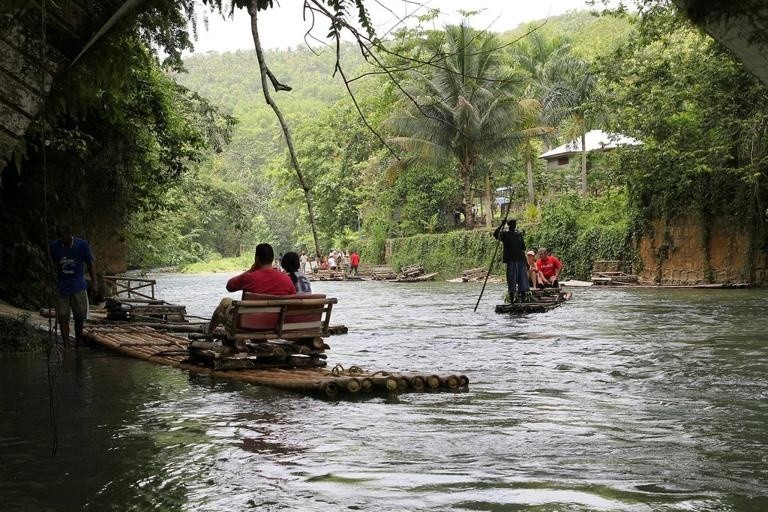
[83,290,470,400]
[557,271,753,289]
[305,269,344,281]
[445,266,503,284]
[360,263,442,282]
[495,281,572,314]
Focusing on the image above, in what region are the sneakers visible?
[538,280,558,290]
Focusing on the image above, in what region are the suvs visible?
[471,188,511,214]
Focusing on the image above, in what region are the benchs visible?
[211,291,338,367]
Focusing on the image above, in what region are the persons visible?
[472,204,479,225]
[45,234,98,353]
[527,247,562,289]
[206,244,360,334]
[493,218,531,303]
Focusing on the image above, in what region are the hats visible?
[527,251,535,255]
